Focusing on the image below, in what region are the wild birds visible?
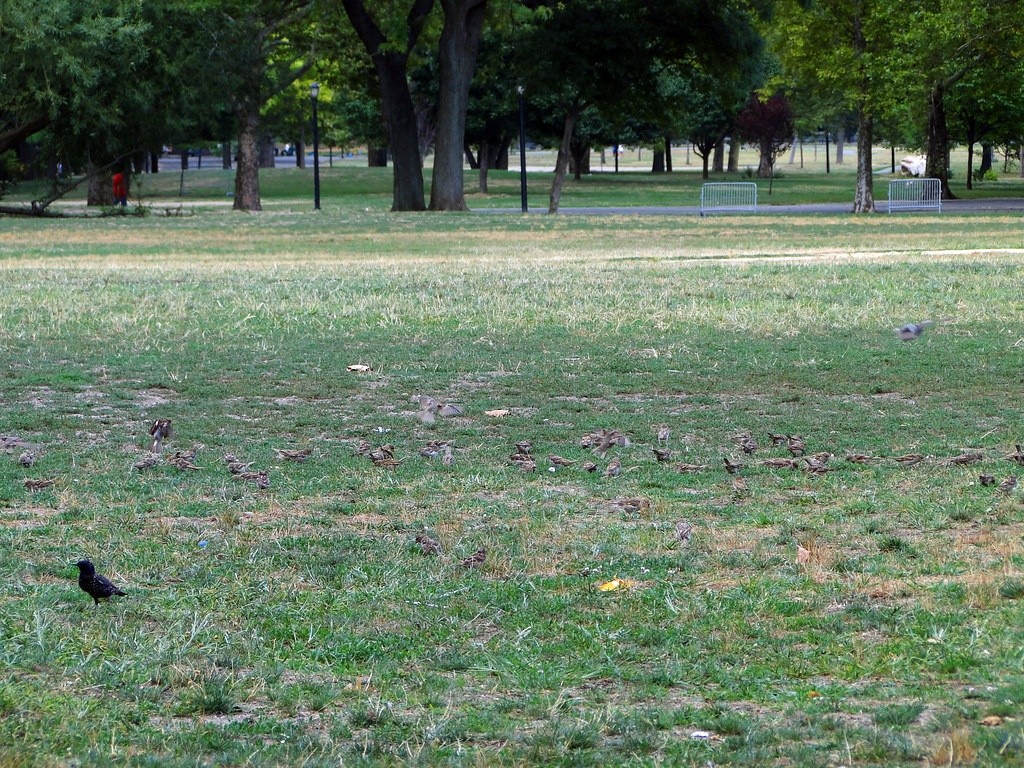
[722,430,1023,495]
[132,417,314,489]
[508,428,708,481]
[70,559,129,607]
[351,440,476,471]
[609,497,652,514]
[412,533,488,568]
[891,315,934,342]
[17,453,56,492]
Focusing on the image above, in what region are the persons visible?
[112,167,127,207]
[612,145,623,159]
[56,159,63,177]
[284,142,289,152]
